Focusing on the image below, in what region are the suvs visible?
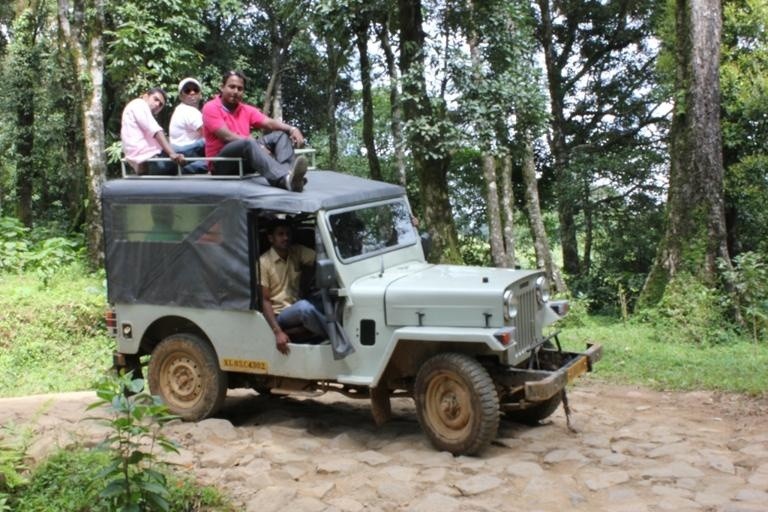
[101,169,602,455]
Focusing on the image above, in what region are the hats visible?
[178,77,202,93]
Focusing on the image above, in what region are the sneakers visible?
[287,156,308,193]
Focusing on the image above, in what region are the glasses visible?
[183,88,200,94]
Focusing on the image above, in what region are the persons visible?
[255,218,328,355]
[145,204,184,241]
[200,70,309,192]
[166,77,204,147]
[364,202,419,252]
[118,86,207,177]
[332,217,364,259]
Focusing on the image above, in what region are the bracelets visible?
[289,126,296,135]
